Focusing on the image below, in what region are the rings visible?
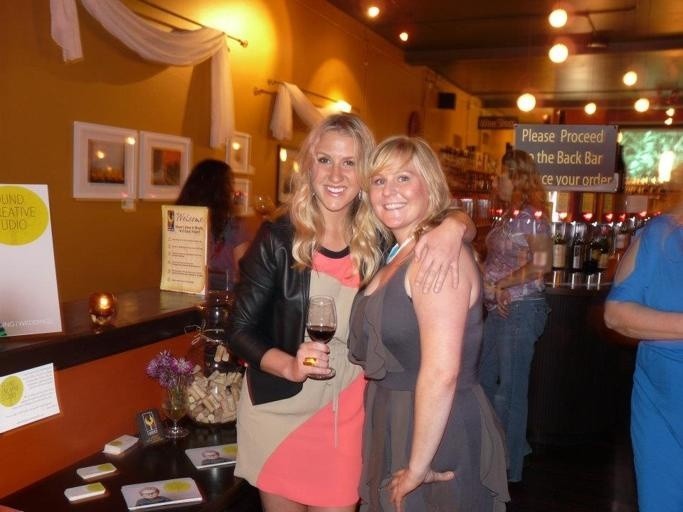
[303,358,316,366]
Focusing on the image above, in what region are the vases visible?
[160,385,190,439]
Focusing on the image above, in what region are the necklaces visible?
[385,236,415,265]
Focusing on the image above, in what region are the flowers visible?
[145,349,198,410]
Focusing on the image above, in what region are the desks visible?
[0,413,245,512]
[0,288,234,501]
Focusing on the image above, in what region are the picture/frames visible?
[73,122,138,201]
[226,177,250,217]
[226,130,252,175]
[277,142,301,203]
[138,130,192,201]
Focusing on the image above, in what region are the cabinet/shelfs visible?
[441,148,497,227]
[528,285,638,459]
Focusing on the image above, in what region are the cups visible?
[307,296,339,379]
[163,387,185,438]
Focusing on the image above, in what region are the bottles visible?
[586,226,600,269]
[614,221,628,261]
[569,223,584,269]
[552,232,568,269]
[601,225,609,269]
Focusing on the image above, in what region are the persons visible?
[481,150,553,489]
[604,163,683,512]
[202,450,231,465]
[346,135,510,512]
[225,112,477,512]
[174,159,270,291]
[136,488,170,507]
